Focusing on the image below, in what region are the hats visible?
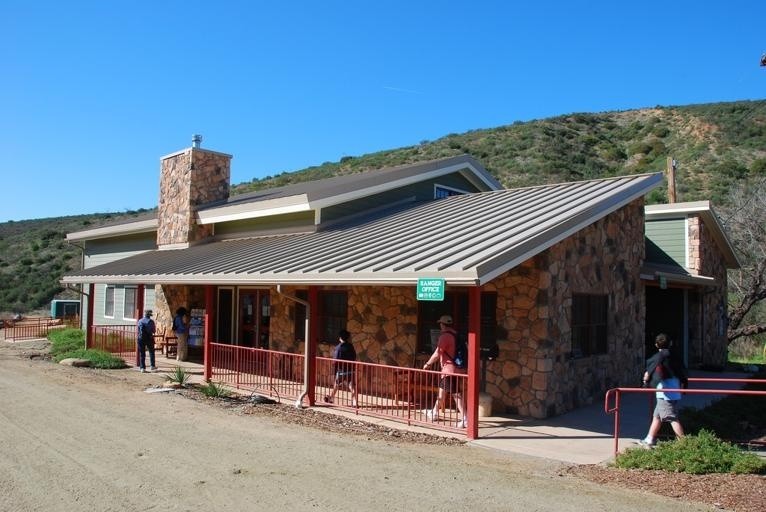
[144,310,153,316]
[437,315,453,324]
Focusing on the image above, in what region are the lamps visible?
[262,304,271,316]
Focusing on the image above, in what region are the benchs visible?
[394,354,467,408]
[146,334,178,357]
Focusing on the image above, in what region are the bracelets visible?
[426,362,432,366]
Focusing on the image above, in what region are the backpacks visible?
[442,330,468,369]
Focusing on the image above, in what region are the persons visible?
[172,306,191,362]
[324,330,358,404]
[637,333,688,446]
[138,309,158,373]
[423,315,467,427]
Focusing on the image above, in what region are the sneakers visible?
[455,417,467,429]
[141,366,158,373]
[423,409,439,420]
